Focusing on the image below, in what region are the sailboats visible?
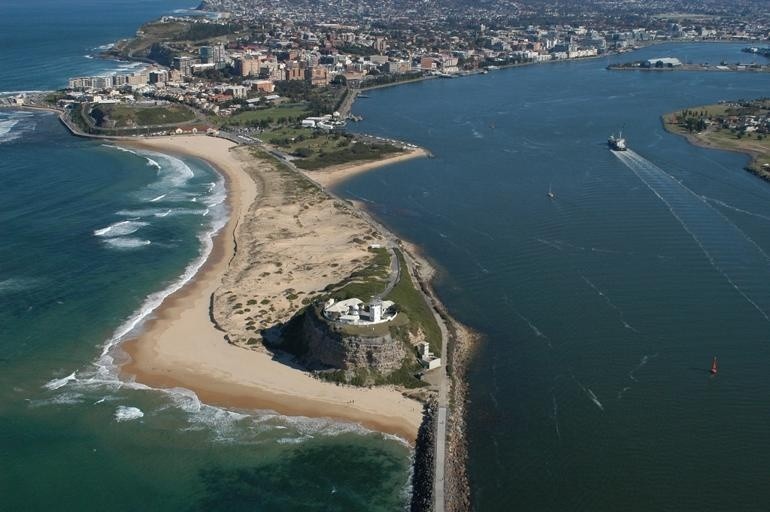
[709,355,718,374]
[547,181,554,198]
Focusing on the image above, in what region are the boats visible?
[608,129,628,152]
